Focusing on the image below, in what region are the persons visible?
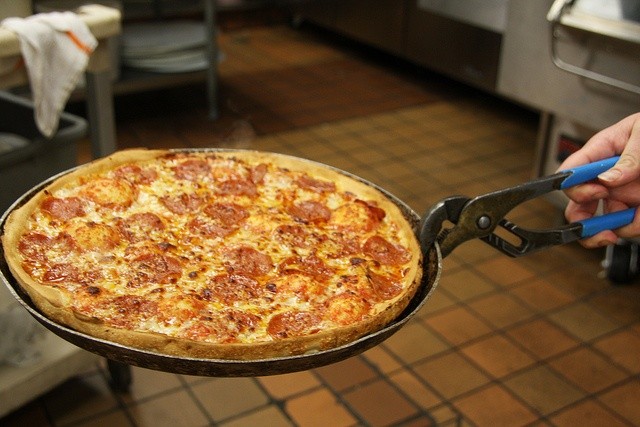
[553,113,640,249]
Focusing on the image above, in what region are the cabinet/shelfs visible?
[0,3,133,418]
[112,1,220,121]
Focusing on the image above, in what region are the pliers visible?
[416,155,635,259]
